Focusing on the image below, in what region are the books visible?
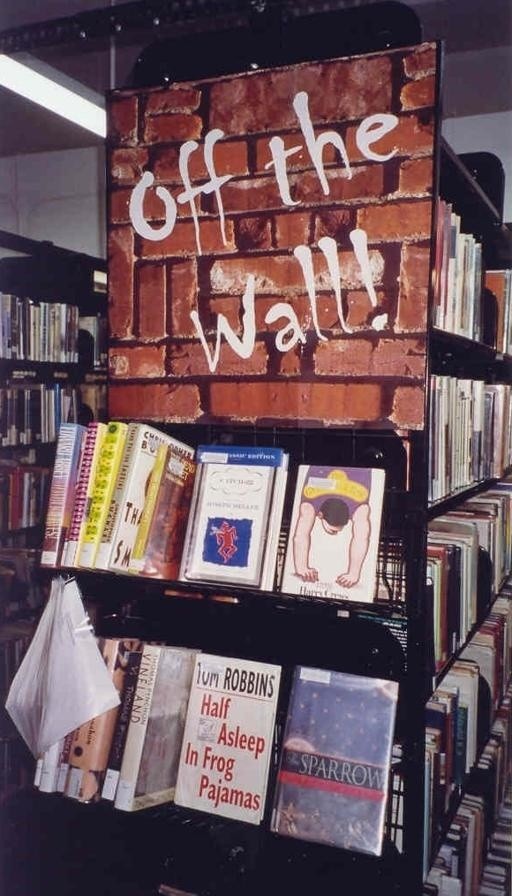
[31,422,397,856]
[1,293,103,809]
[422,194,512,896]
